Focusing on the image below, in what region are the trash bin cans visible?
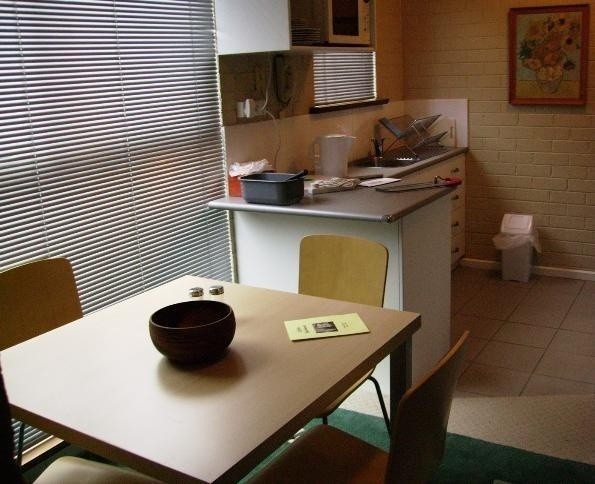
[499,214,537,282]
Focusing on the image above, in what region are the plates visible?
[291,17,322,44]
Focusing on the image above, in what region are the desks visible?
[1,274,423,484]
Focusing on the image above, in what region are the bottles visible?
[189,287,227,306]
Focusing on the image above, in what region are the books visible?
[283,311,370,341]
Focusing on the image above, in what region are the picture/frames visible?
[508,2,591,106]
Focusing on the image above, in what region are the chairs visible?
[34,456,160,482]
[1,257,84,353]
[247,330,470,483]
[291,234,393,439]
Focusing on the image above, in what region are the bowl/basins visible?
[149,300,237,363]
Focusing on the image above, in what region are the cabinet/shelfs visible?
[397,152,467,270]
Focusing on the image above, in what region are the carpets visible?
[20,407,595,484]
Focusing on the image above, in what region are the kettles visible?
[311,134,358,178]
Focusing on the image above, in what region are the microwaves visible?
[325,0,371,48]
